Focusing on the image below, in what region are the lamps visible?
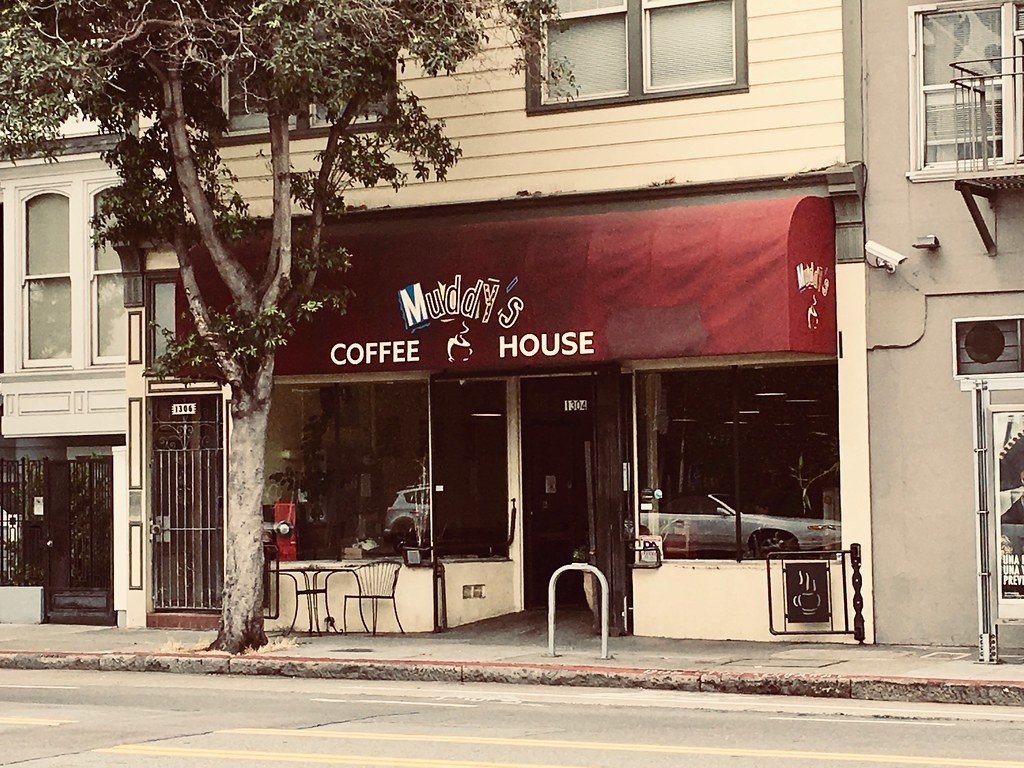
[912,234,939,248]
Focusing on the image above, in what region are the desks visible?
[267,568,351,637]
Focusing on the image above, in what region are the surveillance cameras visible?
[865,241,908,266]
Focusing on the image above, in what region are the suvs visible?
[386,484,429,556]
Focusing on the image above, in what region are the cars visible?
[639,490,842,560]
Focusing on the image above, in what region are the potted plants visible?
[571,545,593,612]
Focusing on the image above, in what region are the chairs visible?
[344,560,407,635]
